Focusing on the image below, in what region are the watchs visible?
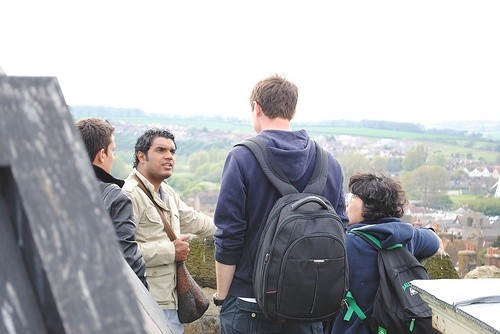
[213,292,227,305]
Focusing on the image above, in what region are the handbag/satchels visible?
[176,260,210,325]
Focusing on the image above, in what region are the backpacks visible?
[348,227,440,334]
[232,136,351,322]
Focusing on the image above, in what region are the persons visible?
[122,129,217,334]
[346,173,441,334]
[77,119,148,290]
[213,77,349,334]
